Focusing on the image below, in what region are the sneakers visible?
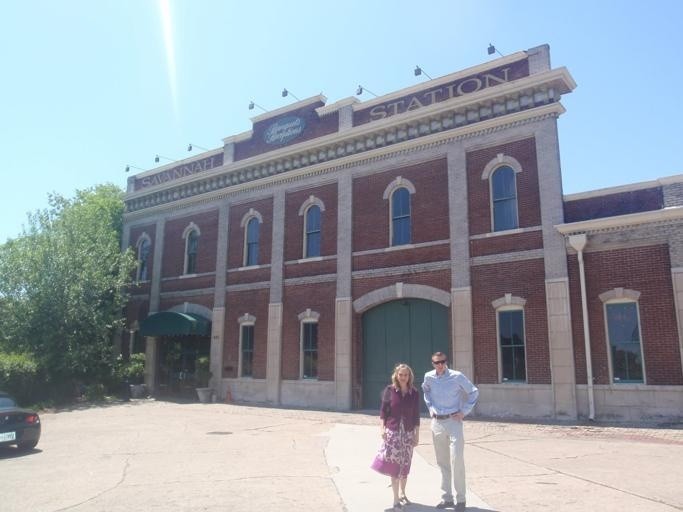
[399,495,410,504]
[394,503,403,512]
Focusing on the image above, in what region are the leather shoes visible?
[455,503,466,512]
[437,502,454,508]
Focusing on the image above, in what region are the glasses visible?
[430,360,448,364]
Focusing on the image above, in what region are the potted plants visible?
[192,356,214,402]
[121,353,147,399]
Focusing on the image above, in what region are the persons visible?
[421,351,479,512]
[371,364,420,512]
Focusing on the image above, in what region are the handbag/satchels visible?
[383,400,390,416]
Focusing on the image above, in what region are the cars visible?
[0,393,42,453]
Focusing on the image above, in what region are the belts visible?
[433,412,459,420]
